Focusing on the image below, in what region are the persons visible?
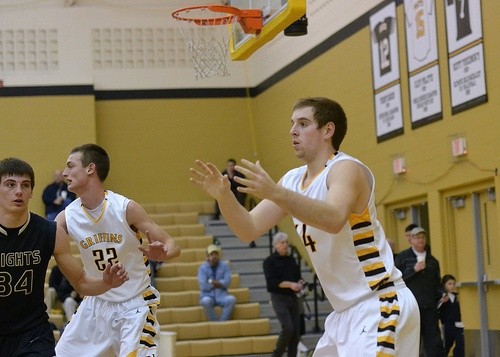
[261,232,306,357]
[41,144,181,357]
[393,223,465,357]
[189,97,421,357]
[0,158,130,357]
[197,244,236,322]
[209,159,248,221]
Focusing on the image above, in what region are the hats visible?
[410,227,427,235]
[206,244,220,255]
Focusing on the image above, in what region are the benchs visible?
[43,203,280,357]
